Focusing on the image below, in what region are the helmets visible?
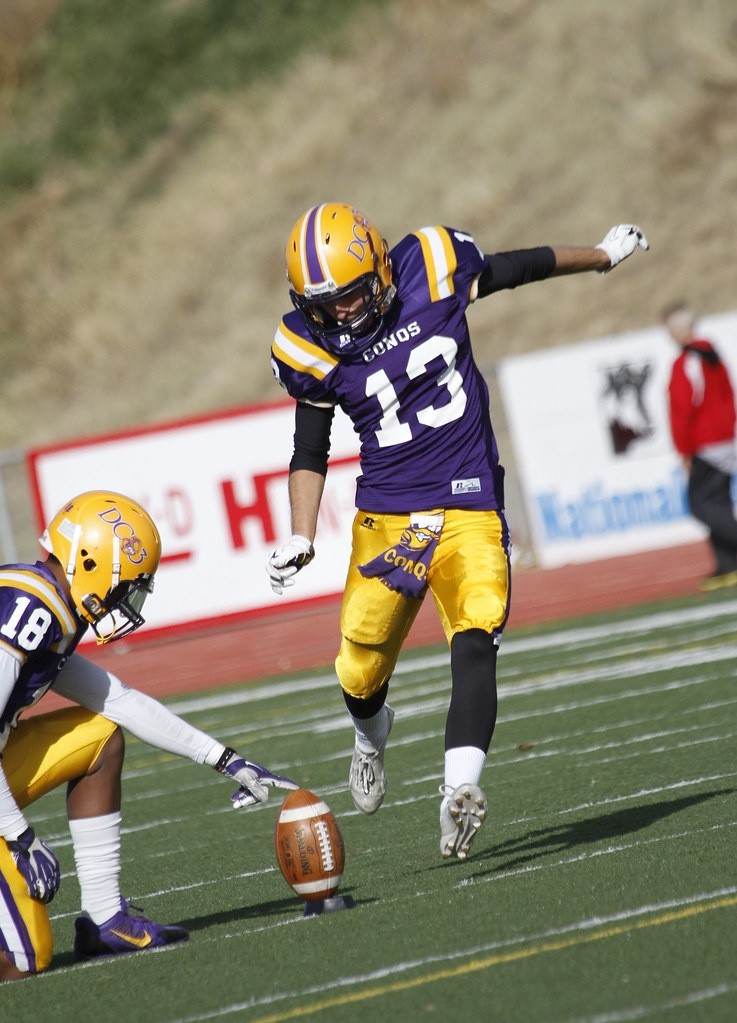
[286,201,396,360]
[38,490,162,646]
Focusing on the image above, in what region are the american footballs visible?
[274,787,345,901]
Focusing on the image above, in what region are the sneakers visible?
[348,702,396,816]
[439,783,487,858]
[75,894,189,961]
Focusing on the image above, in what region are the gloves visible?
[221,751,299,810]
[264,533,315,593]
[6,826,61,905]
[594,223,648,272]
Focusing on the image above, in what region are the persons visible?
[662,300,737,592]
[268,203,648,859]
[0,491,301,983]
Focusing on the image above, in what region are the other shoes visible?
[699,569,737,592]
